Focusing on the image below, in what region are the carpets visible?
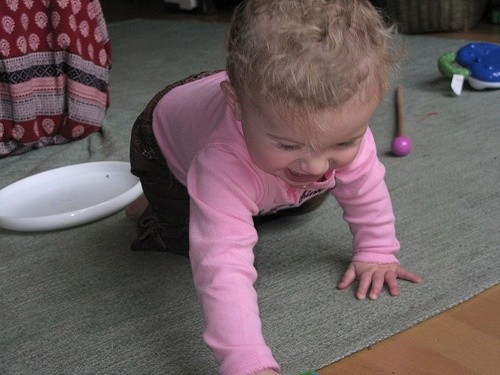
[0,18,500,375]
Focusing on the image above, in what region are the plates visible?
[0,159,143,231]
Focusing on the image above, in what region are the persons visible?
[126,0,422,375]
